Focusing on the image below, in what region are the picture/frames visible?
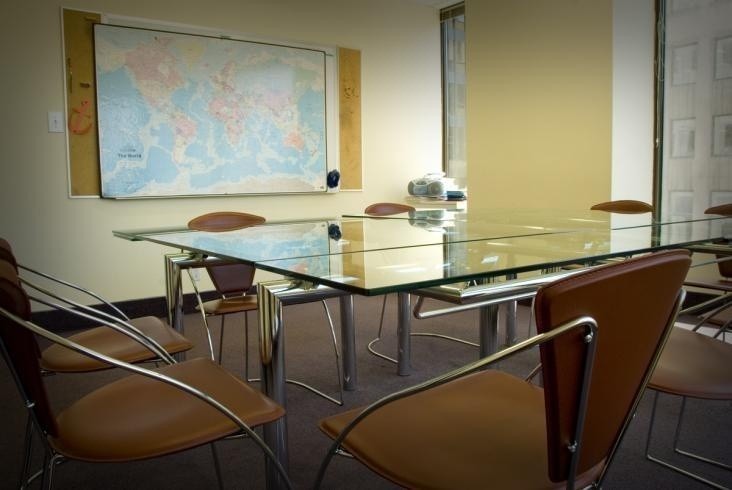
[669,36,731,238]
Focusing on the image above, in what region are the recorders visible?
[408,178,445,196]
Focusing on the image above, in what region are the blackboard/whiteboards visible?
[101,11,340,200]
